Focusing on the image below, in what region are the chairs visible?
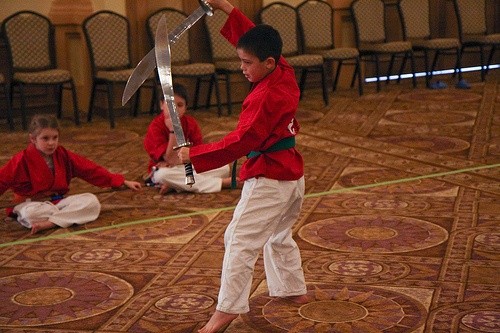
[146,7,221,118]
[257,3,330,107]
[396,0,463,88]
[82,10,161,129]
[350,0,416,92]
[205,8,254,117]
[296,0,363,96]
[453,0,500,82]
[0,10,81,131]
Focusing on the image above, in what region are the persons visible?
[143,82,242,194]
[0,112,141,235]
[177,0,308,333]
